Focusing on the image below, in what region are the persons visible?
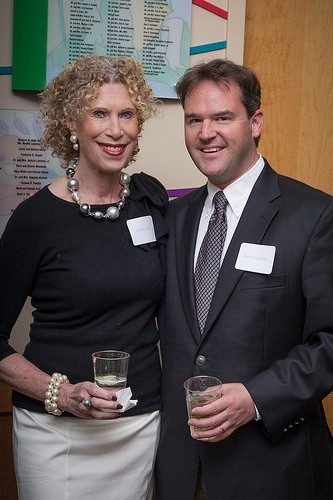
[152,59,333,500]
[0,56,172,500]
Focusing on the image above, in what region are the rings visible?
[219,425,226,433]
[82,395,93,408]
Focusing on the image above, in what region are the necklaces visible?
[66,159,131,221]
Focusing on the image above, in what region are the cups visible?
[184,376,222,440]
[92,351,130,392]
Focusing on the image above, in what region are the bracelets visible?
[44,372,69,416]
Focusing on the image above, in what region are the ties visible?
[193,191,229,336]
